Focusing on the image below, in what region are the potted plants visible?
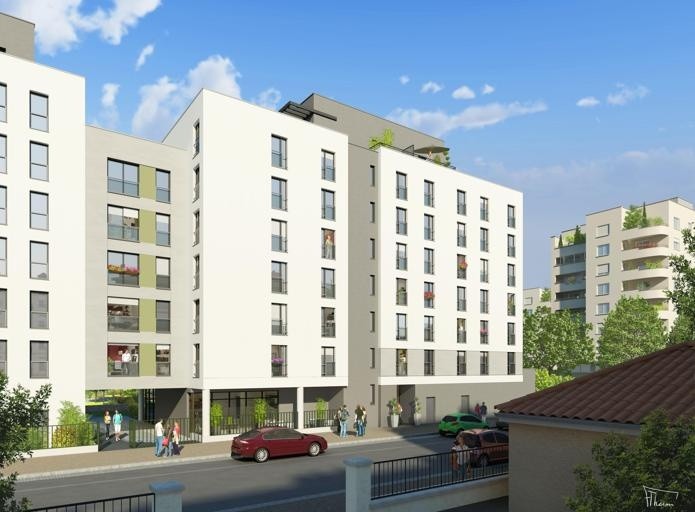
[387,397,403,428]
[412,397,423,426]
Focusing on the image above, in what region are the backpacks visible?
[340,408,348,421]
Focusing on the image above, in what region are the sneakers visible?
[105,437,120,442]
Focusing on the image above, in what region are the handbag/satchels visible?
[466,465,472,479]
[162,439,169,446]
